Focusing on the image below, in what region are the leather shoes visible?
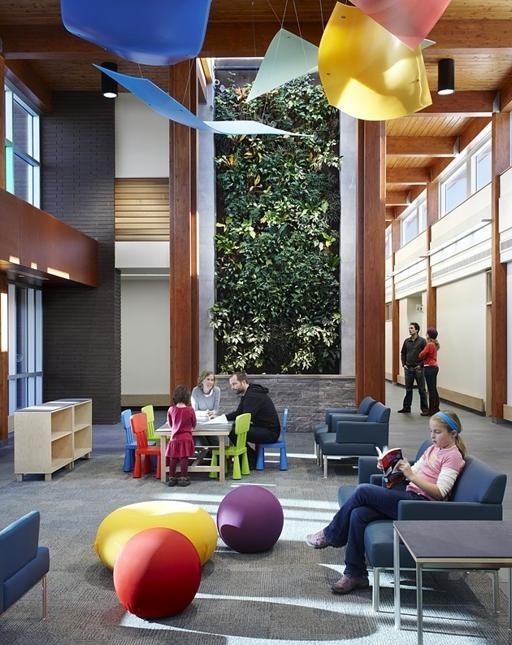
[398,408,411,413]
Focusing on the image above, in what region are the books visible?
[375,446,403,489]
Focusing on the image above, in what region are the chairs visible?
[139,405,168,444]
[121,409,150,472]
[208,413,249,479]
[131,412,161,480]
[255,408,289,471]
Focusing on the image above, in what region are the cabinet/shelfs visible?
[14,403,75,481]
[53,398,92,466]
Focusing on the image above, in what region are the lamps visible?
[102,62,117,99]
[435,58,453,95]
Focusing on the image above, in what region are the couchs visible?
[337,440,507,614]
[314,396,375,462]
[0,509,50,625]
[319,402,390,478]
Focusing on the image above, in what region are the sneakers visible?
[330,571,370,596]
[306,530,347,549]
[167,476,177,487]
[178,476,191,487]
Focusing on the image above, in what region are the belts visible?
[424,364,437,367]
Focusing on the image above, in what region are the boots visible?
[420,396,440,417]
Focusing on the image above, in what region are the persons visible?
[307,409,465,594]
[191,369,220,466]
[166,384,196,486]
[418,327,440,416]
[225,372,280,470]
[398,322,428,413]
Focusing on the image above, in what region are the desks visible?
[156,410,234,483]
[392,520,512,644]
